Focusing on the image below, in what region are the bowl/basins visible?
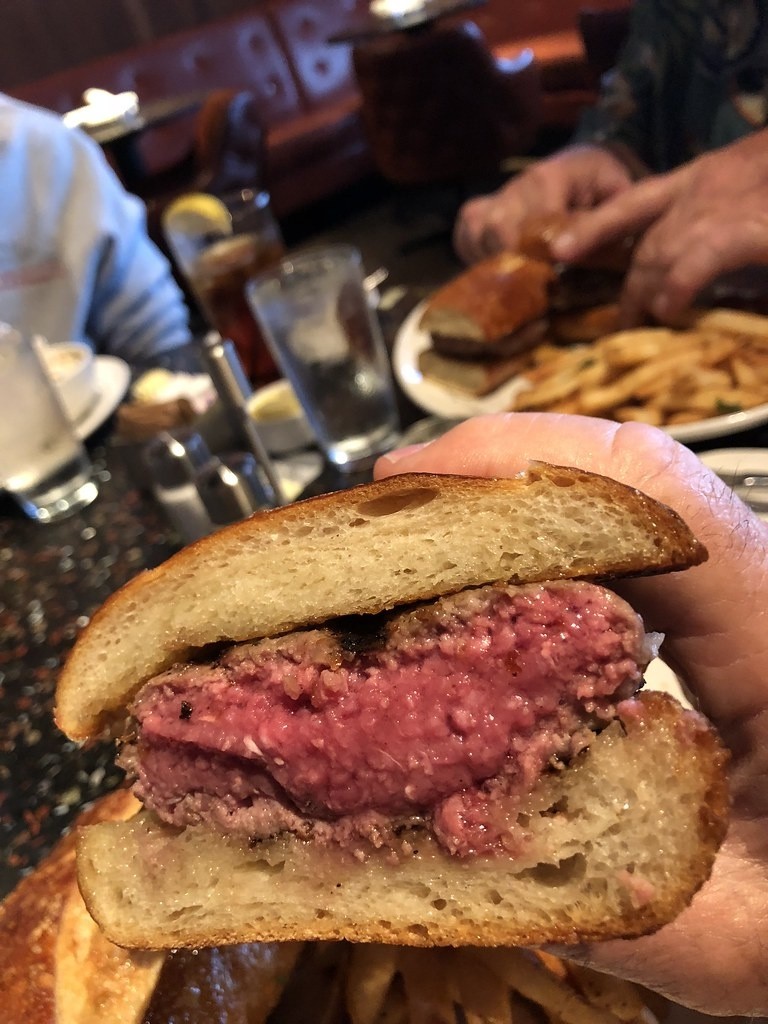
[43,342,94,422]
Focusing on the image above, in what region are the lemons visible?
[246,378,306,421]
[165,192,232,237]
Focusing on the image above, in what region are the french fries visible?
[344,943,651,1024]
[491,301,768,429]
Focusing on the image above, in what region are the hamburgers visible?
[53,459,732,953]
[526,211,655,341]
[413,248,559,397]
[0,782,358,1024]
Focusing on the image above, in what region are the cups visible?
[247,242,398,467]
[0,327,98,520]
[170,188,289,389]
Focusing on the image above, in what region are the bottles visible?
[195,452,274,530]
[144,438,218,540]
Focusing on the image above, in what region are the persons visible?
[0,90,191,361]
[454,0,767,326]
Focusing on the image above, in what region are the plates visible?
[392,289,768,440]
[77,355,130,439]
